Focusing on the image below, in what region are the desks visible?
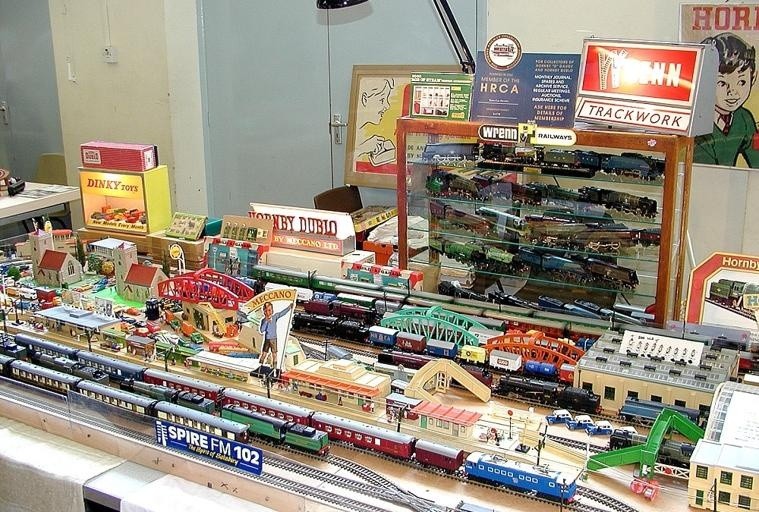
[0,177,81,238]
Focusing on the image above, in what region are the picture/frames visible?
[678,1,759,173]
[344,63,468,192]
[685,252,759,344]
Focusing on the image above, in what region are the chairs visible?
[28,153,70,229]
[314,185,365,221]
